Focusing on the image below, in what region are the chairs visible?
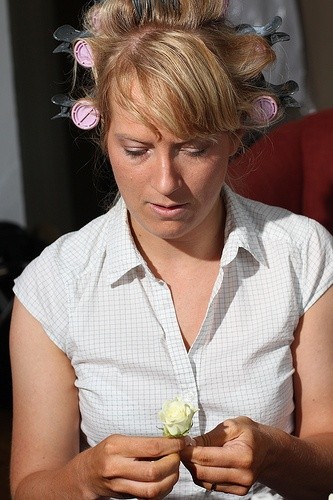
[224,105,333,237]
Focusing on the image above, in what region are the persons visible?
[9,0,333,500]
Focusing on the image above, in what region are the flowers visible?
[155,396,202,439]
